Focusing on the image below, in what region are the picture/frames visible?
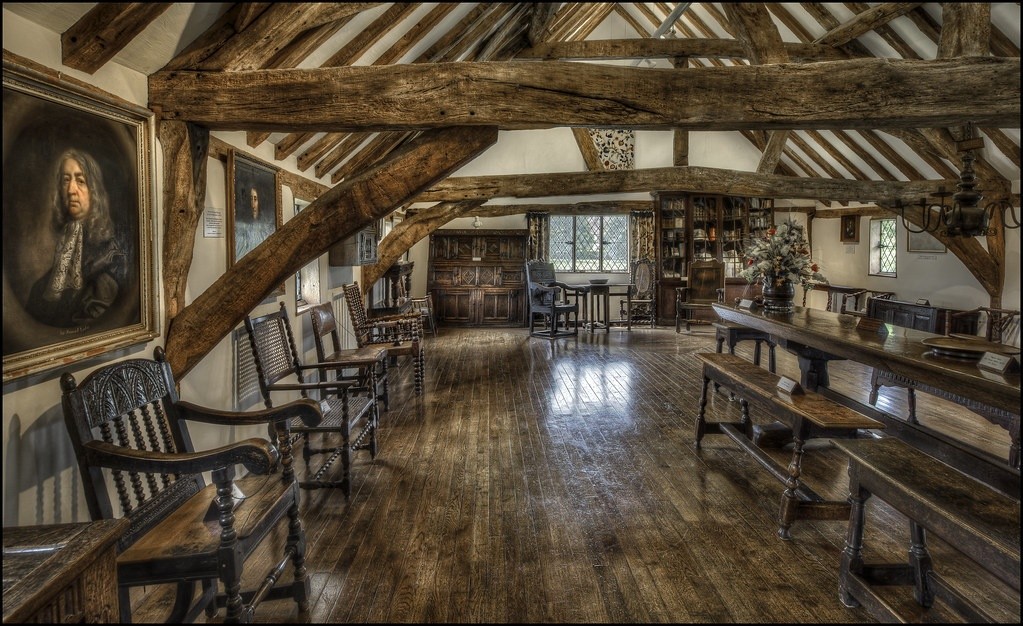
[0,48,161,381]
[840,215,860,242]
[227,147,286,297]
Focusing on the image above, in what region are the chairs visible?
[412,292,438,338]
[61,345,324,623]
[842,290,895,319]
[525,258,590,340]
[310,302,391,420]
[945,306,1020,346]
[245,302,382,497]
[676,260,725,334]
[343,281,425,396]
[620,258,656,330]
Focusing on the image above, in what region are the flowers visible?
[743,225,826,288]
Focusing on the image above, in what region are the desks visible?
[802,283,866,311]
[2,519,132,624]
[564,284,635,333]
[712,301,1020,468]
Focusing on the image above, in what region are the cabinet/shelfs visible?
[656,194,774,326]
[426,229,529,329]
[871,299,980,335]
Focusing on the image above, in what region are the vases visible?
[763,280,795,314]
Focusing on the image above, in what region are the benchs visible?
[830,436,1020,623]
[869,368,1020,470]
[695,352,887,540]
[713,323,776,373]
